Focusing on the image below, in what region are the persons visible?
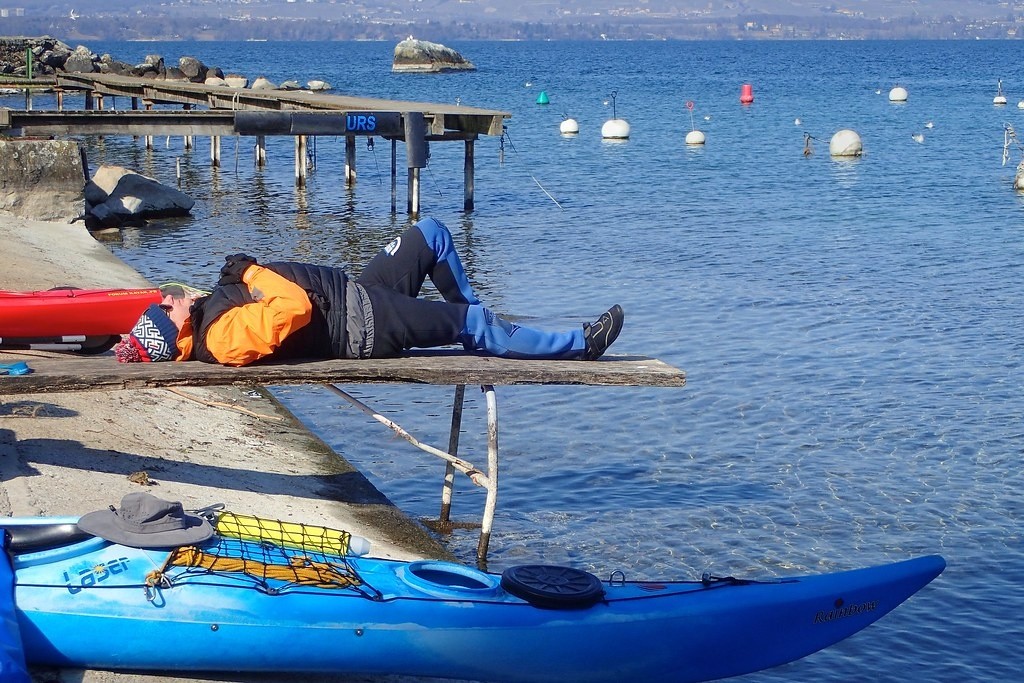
[115,217,625,368]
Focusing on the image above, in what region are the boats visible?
[0,509,948,683]
[0,285,182,341]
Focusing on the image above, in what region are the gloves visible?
[218,253,256,286]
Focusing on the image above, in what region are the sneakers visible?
[580,304,624,360]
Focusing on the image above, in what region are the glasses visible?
[159,304,174,317]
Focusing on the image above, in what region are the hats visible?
[115,304,179,363]
[77,491,213,549]
